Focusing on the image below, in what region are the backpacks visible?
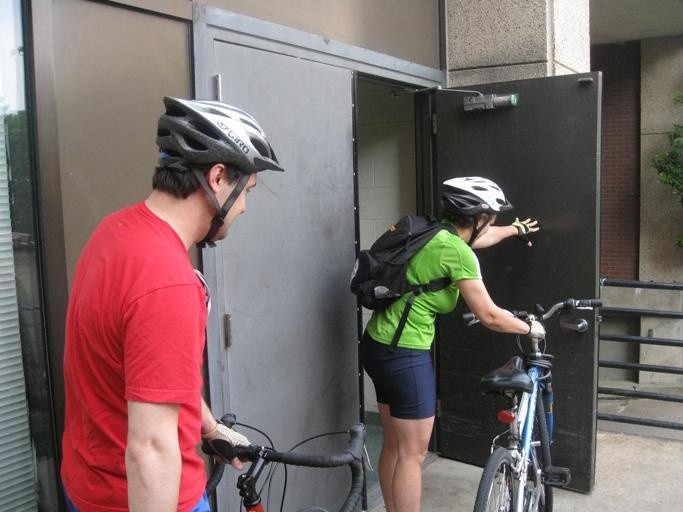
[349,212,460,312]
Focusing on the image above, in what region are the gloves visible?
[510,220,534,246]
[525,320,547,342]
[200,418,252,464]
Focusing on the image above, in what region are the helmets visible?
[155,95,286,176]
[438,176,514,217]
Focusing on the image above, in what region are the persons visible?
[356,174,551,512]
[60,96,286,512]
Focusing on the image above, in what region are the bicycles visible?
[198,412,382,512]
[462,293,606,511]
